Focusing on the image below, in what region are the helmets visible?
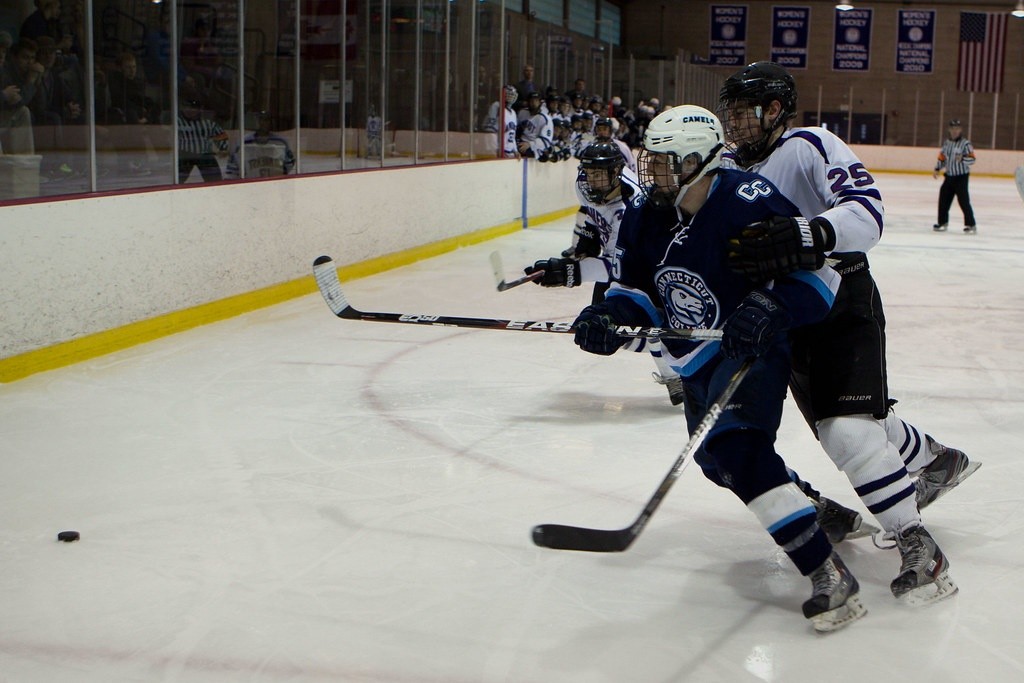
[714,60,798,161]
[636,104,725,210]
[254,103,271,120]
[575,140,626,202]
[502,85,671,132]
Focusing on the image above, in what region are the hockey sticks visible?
[489,251,586,293]
[310,250,724,343]
[368,120,392,148]
[637,144,646,160]
[529,356,757,554]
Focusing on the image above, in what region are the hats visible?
[194,18,211,28]
[949,119,961,127]
[183,96,199,109]
[37,36,58,50]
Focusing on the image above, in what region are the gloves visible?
[525,257,582,289]
[726,216,825,280]
[573,294,644,355]
[719,288,794,359]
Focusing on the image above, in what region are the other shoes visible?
[963,225,976,234]
[933,223,946,231]
[41,160,170,183]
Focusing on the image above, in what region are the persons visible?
[0,0,295,182]
[718,61,983,609]
[572,105,881,632]
[365,108,381,160]
[523,140,684,410]
[480,66,671,163]
[933,120,976,235]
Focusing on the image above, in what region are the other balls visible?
[58,530,80,542]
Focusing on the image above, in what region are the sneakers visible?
[910,433,980,508]
[665,375,686,407]
[815,494,862,544]
[801,551,868,635]
[890,527,957,608]
